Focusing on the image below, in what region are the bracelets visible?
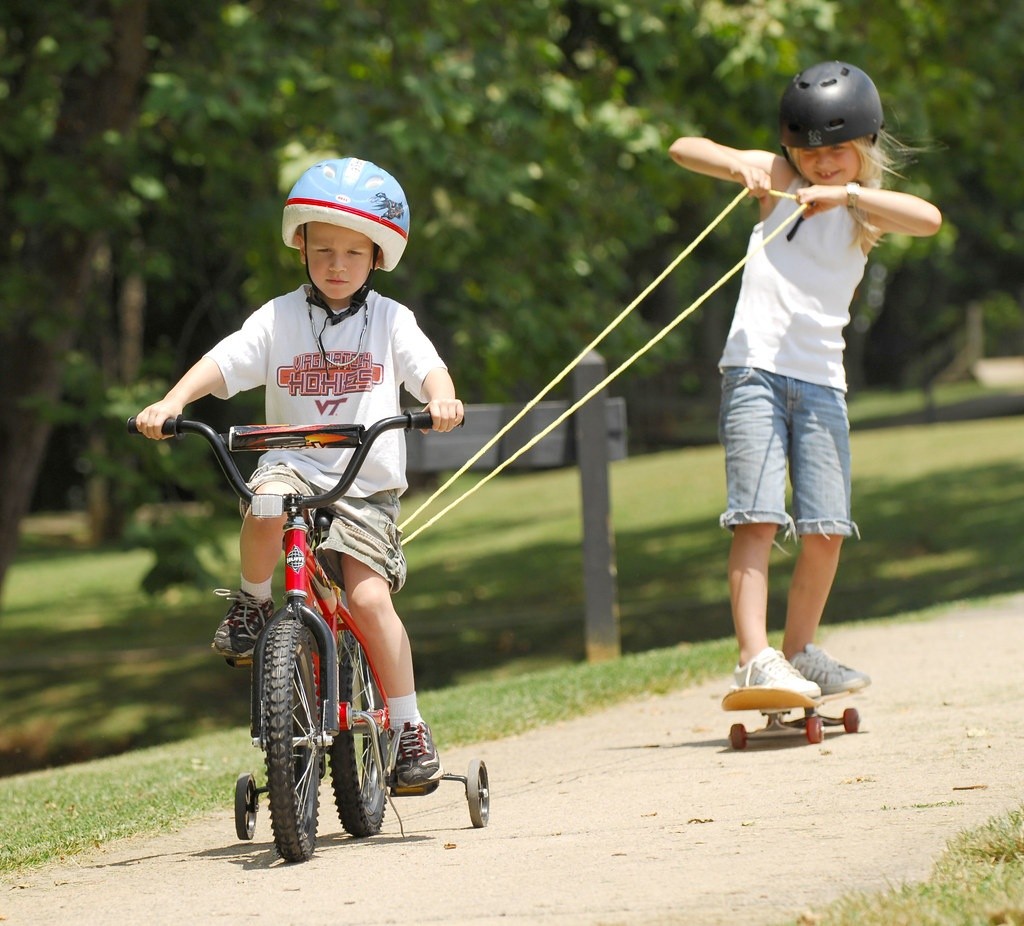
[846,182,858,207]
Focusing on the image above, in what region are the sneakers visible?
[734,647,822,699]
[381,722,442,788]
[211,588,275,658]
[788,644,869,693]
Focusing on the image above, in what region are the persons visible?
[136,157,464,788]
[666,60,942,698]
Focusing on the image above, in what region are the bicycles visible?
[126,412,491,868]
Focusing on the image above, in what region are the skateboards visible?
[722,689,861,749]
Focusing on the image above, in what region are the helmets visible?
[779,59,884,149]
[282,157,410,271]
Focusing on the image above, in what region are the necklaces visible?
[308,289,368,366]
